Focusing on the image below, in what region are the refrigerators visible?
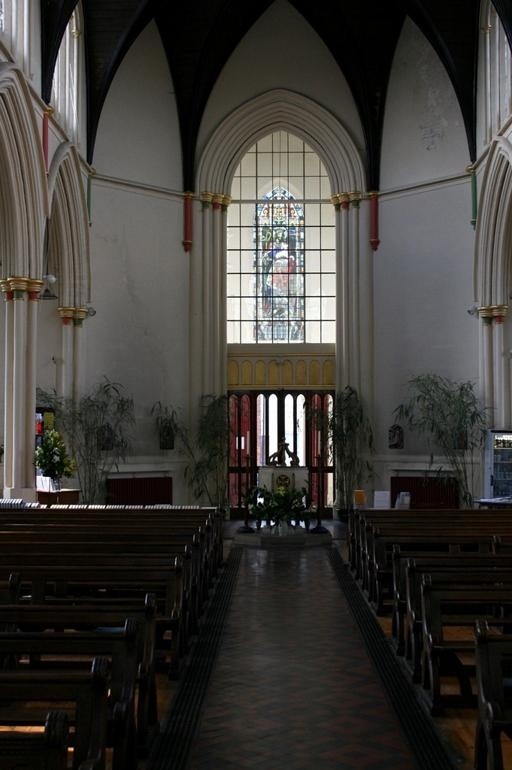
[486,428,512,498]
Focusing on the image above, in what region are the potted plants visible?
[32,427,78,491]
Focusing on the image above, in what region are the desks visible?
[37,488,81,505]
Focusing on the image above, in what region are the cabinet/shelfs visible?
[491,430,512,500]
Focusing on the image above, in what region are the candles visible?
[247,429,250,456]
[318,431,321,456]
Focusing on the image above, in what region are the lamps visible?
[38,216,58,300]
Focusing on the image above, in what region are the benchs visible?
[340,504,511,770]
[1,493,228,767]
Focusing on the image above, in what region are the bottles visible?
[396,495,400,509]
[493,435,512,448]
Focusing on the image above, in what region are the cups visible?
[400,492,410,509]
